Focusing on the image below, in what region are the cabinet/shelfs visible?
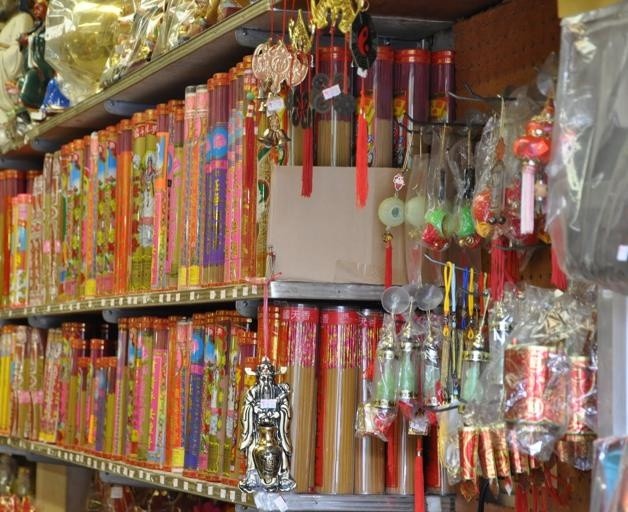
[0,1,457,512]
